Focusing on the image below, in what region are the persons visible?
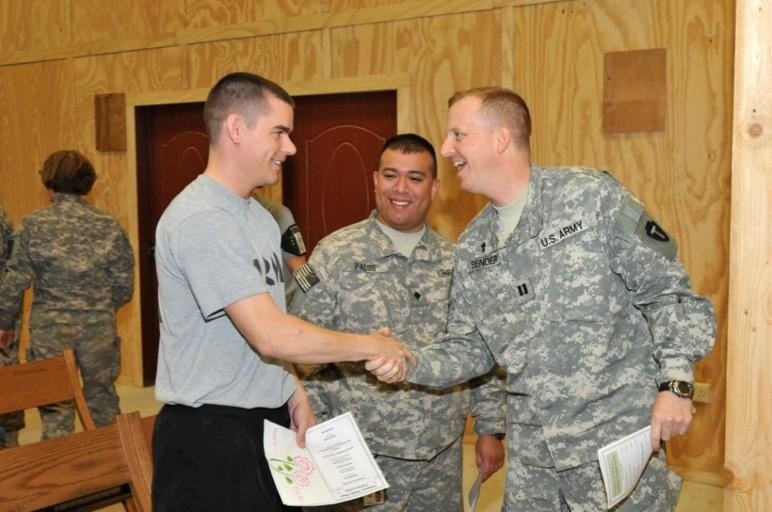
[150,71,416,510]
[286,134,505,512]
[365,86,718,512]
[251,183,305,285]
[0,206,26,450]
[0,150,134,439]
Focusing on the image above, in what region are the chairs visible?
[0,347,98,446]
[0,411,152,512]
[129,408,160,492]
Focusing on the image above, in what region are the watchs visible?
[658,381,694,400]
